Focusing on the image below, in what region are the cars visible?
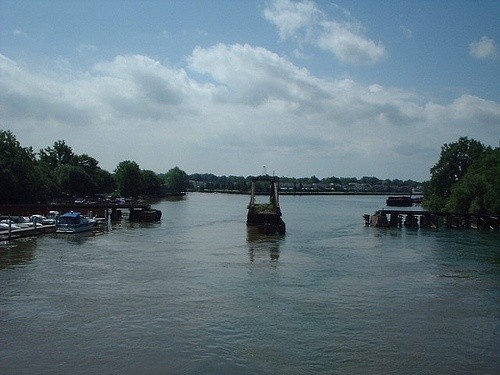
[1,210,59,229]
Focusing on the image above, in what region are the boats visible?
[56,210,93,234]
[387,197,411,207]
[248,175,285,232]
